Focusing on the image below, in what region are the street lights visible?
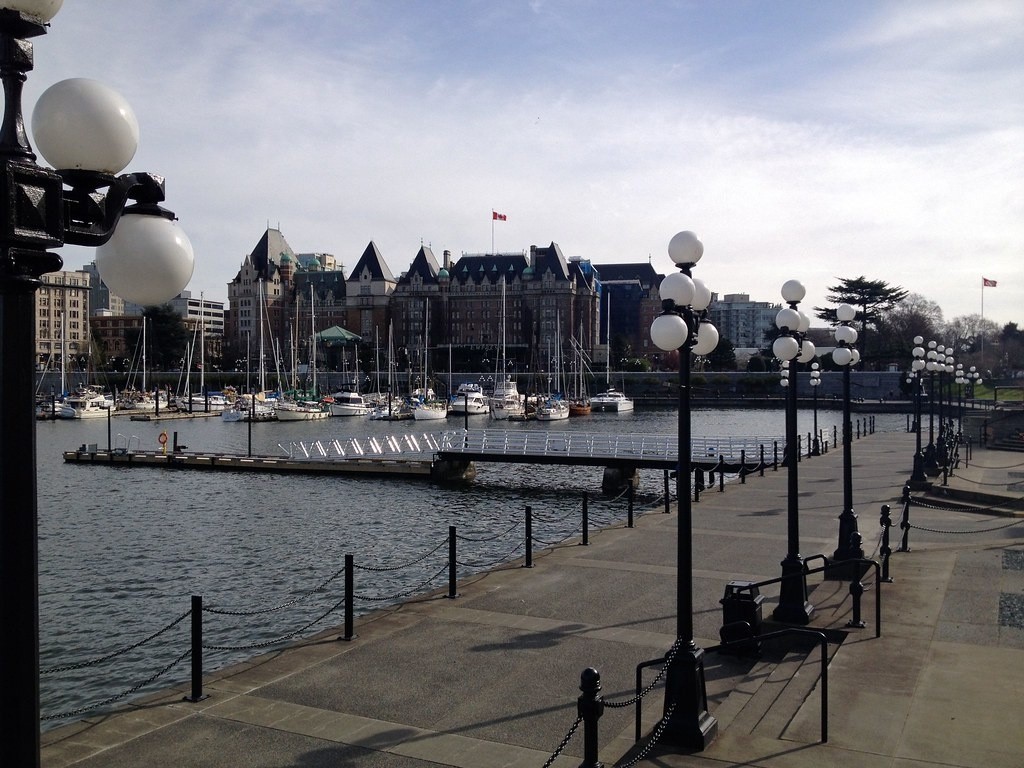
[647,229,722,753]
[770,276,818,624]
[925,341,941,477]
[936,345,950,465]
[954,362,964,441]
[830,301,866,566]
[945,346,955,439]
[911,334,927,482]
[965,366,983,411]
[0,0,196,768]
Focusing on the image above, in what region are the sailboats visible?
[37,273,636,425]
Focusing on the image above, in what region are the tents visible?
[309,326,362,371]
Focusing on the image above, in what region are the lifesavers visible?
[158,433,168,444]
[235,406,239,411]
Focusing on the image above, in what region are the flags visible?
[493,212,506,221]
[984,278,997,287]
